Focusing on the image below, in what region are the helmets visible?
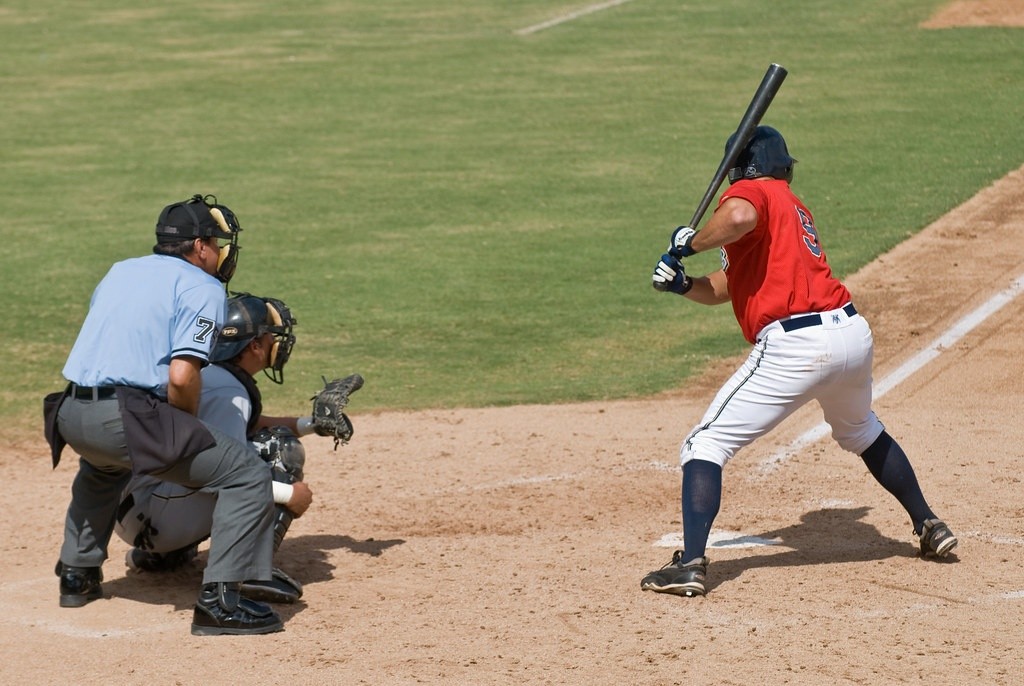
[209,295,274,362]
[725,126,799,183]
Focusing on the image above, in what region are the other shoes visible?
[125,547,155,570]
[241,575,299,604]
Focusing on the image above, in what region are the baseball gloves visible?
[311,373,364,440]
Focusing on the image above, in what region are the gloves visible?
[667,225,700,260]
[652,254,692,294]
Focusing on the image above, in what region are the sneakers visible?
[191,586,283,637]
[641,550,710,597]
[58,564,103,607]
[913,518,958,558]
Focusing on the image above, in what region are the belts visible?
[68,383,115,399]
[781,303,858,332]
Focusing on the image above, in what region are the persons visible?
[41,193,364,637]
[639,126,959,595]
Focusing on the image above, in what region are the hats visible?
[156,201,232,240]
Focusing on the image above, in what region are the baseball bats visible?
[654,62,789,291]
